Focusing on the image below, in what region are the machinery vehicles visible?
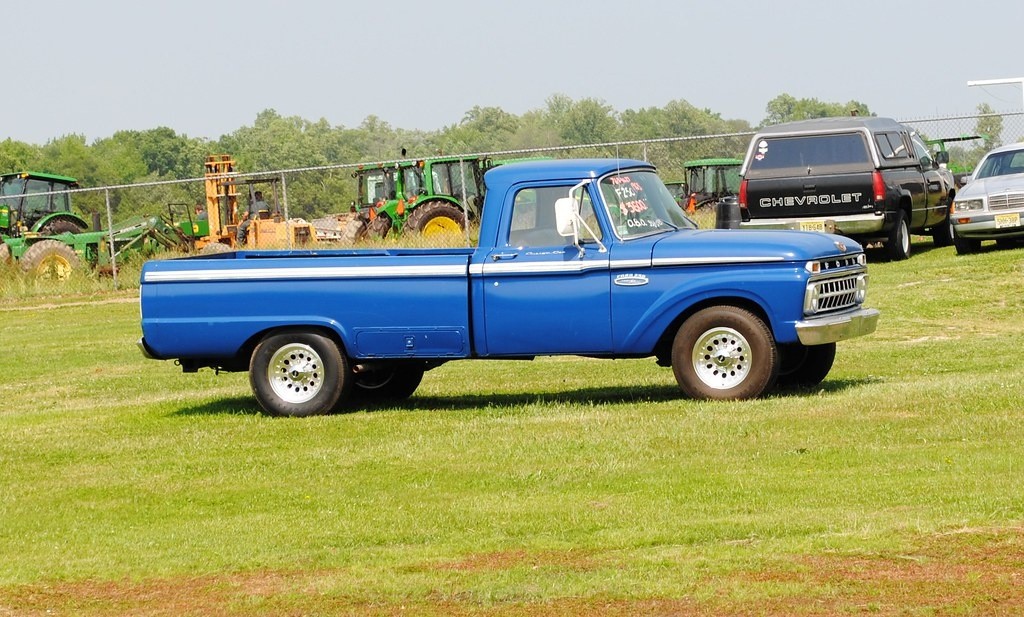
[0,172,210,287]
[349,153,556,249]
[142,155,319,251]
[658,158,745,232]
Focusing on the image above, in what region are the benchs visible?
[517,229,566,247]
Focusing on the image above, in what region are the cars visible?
[949,142,1024,255]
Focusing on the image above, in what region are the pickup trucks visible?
[738,117,956,263]
[137,158,881,420]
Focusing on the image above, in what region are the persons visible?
[236,190,270,241]
[196,205,208,220]
[582,206,620,239]
[238,212,249,226]
